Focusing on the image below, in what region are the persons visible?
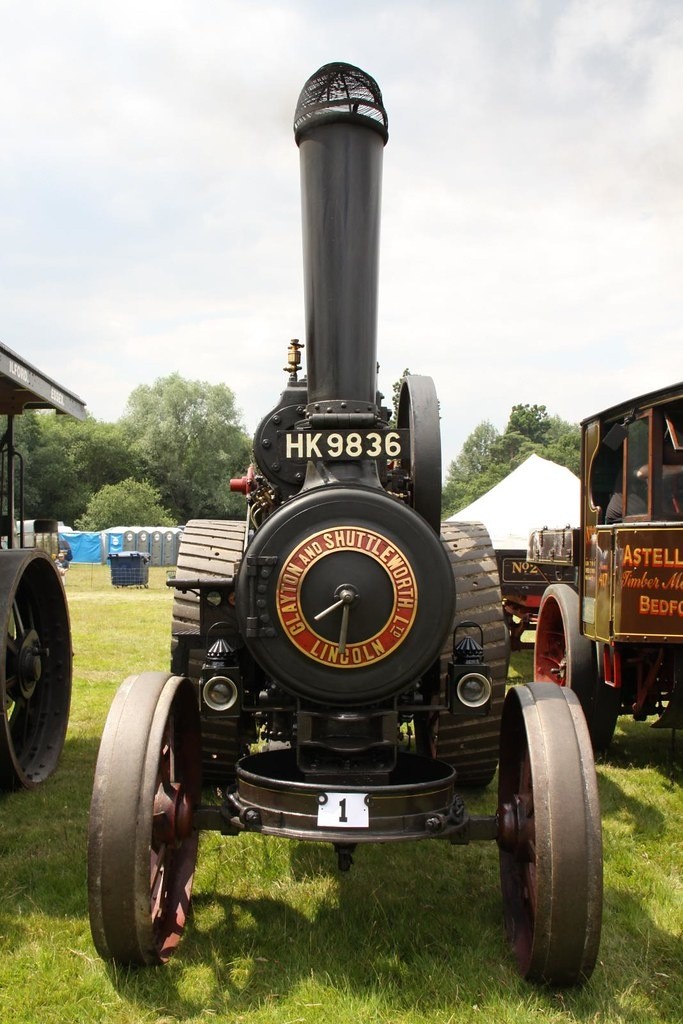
[51,553,70,576]
